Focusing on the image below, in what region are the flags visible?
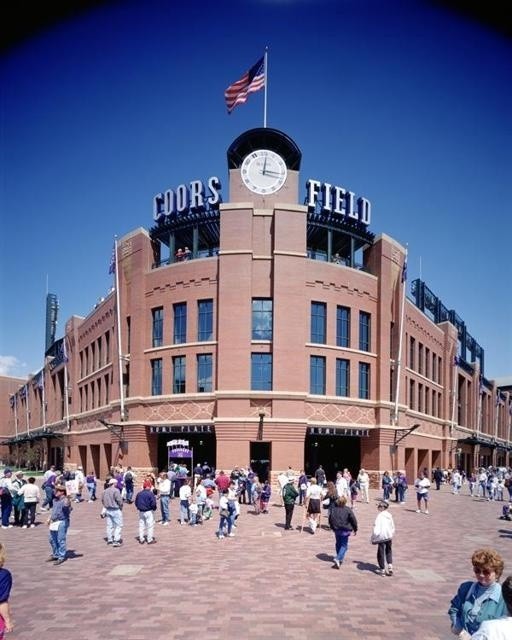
[35,374,44,388]
[454,342,459,364]
[496,391,500,406]
[10,395,17,406]
[508,403,512,415]
[401,260,407,283]
[56,342,68,363]
[223,53,264,115]
[479,378,484,394]
[20,386,28,399]
[109,242,116,274]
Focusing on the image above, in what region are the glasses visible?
[473,567,495,576]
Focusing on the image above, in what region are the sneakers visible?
[54,560,62,565]
[219,521,237,538]
[376,563,394,577]
[415,508,430,514]
[1,523,36,529]
[333,557,341,568]
[46,555,54,562]
[108,536,156,547]
[156,519,204,525]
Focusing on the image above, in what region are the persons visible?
[371,501,395,576]
[414,468,430,514]
[432,465,511,501]
[448,549,512,640]
[333,253,341,263]
[176,247,191,260]
[381,470,408,503]
[1,546,13,639]
[46,486,72,565]
[328,497,358,568]
[0,465,370,547]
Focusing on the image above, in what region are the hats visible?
[288,477,296,483]
[207,490,213,496]
[56,486,64,490]
[107,479,118,485]
[143,482,152,487]
[376,500,389,508]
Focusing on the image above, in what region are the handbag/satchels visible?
[221,509,231,518]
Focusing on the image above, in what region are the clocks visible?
[240,149,288,197]
